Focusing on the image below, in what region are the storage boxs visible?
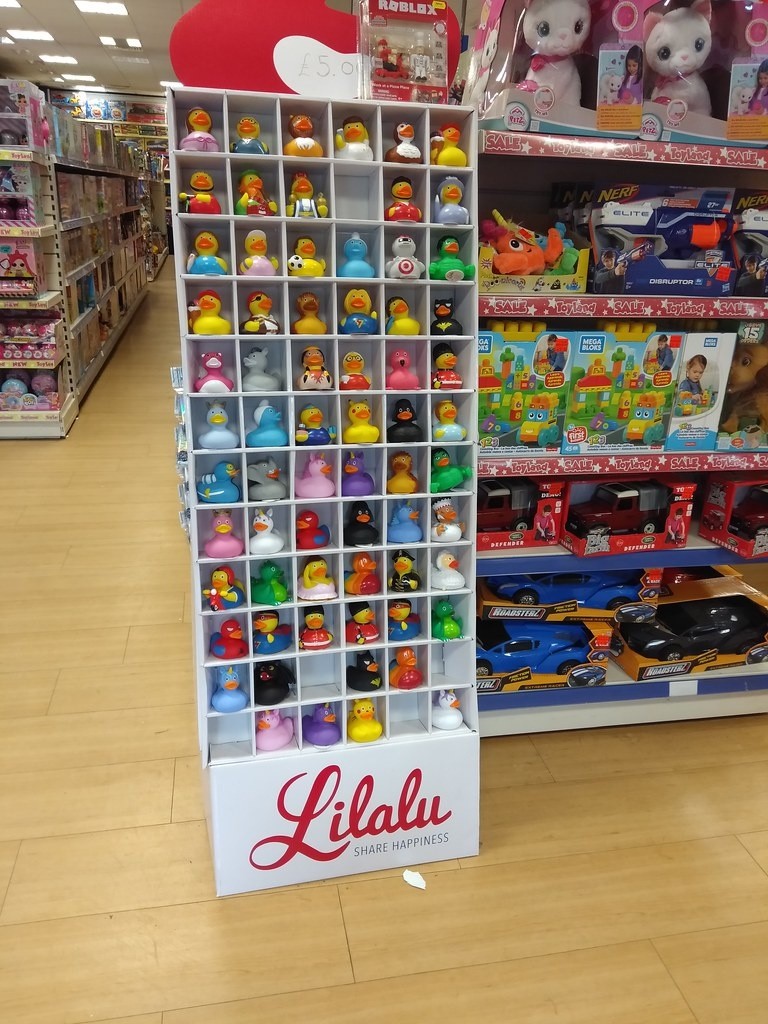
[475,181,768,297]
[479,320,768,450]
[561,470,696,561]
[478,568,665,623]
[474,621,614,692]
[698,473,768,561]
[476,477,561,551]
[468,0,768,149]
[359,0,449,104]
[609,576,768,684]
[663,565,742,587]
[0,76,165,360]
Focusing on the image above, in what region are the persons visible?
[547,334,565,372]
[656,334,674,371]
[378,39,400,73]
[596,251,627,294]
[737,255,767,296]
[748,59,768,114]
[666,508,685,543]
[618,45,643,103]
[411,46,430,80]
[679,354,707,403]
[535,504,554,540]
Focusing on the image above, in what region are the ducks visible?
[198,400,468,448]
[179,106,467,166]
[193,343,464,392]
[294,450,471,499]
[197,461,241,503]
[187,229,476,282]
[202,549,464,749]
[188,288,464,336]
[179,169,470,226]
[204,497,466,558]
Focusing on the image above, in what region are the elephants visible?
[248,456,286,501]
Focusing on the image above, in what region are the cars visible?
[476,614,591,678]
[487,570,642,612]
[621,596,768,661]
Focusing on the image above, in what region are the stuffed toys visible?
[644,0,712,116]
[523,0,590,105]
[483,219,579,275]
[723,344,768,432]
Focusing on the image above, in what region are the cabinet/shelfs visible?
[164,85,487,896]
[141,175,168,282]
[0,149,145,439]
[73,118,174,254]
[478,129,768,738]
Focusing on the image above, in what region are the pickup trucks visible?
[565,481,673,540]
[724,484,768,541]
[477,477,535,531]
